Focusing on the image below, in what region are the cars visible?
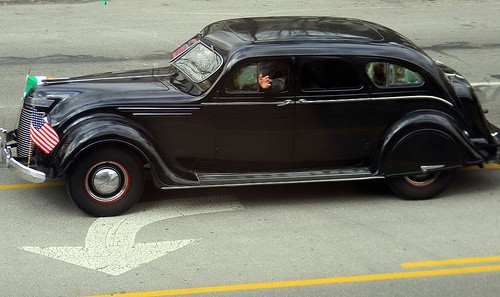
[1,17,500,219]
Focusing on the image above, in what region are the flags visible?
[22,73,46,101]
[30,109,60,156]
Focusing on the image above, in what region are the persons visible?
[258,61,286,93]
